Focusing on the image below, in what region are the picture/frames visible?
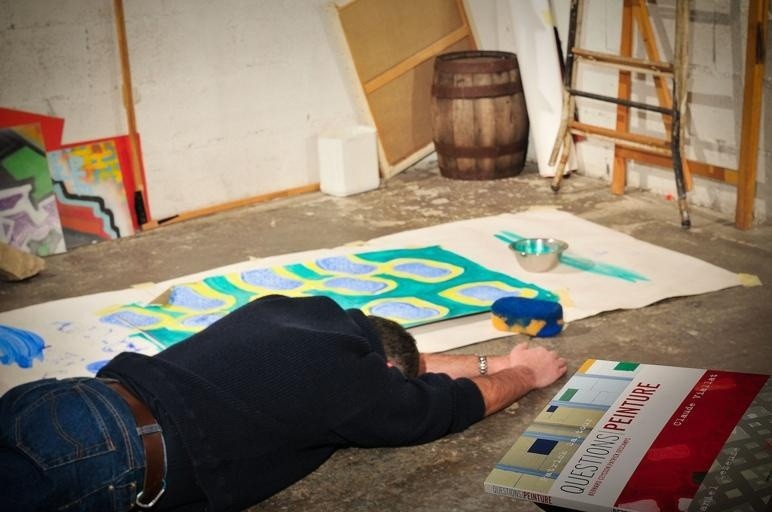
[323,0,483,179]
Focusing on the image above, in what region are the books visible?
[485,358,771,512]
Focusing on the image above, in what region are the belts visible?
[107,380,165,512]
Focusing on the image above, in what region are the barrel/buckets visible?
[430,50,529,180]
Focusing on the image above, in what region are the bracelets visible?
[476,354,491,377]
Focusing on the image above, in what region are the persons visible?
[0,293,567,512]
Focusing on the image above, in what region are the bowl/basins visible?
[509,238,569,270]
